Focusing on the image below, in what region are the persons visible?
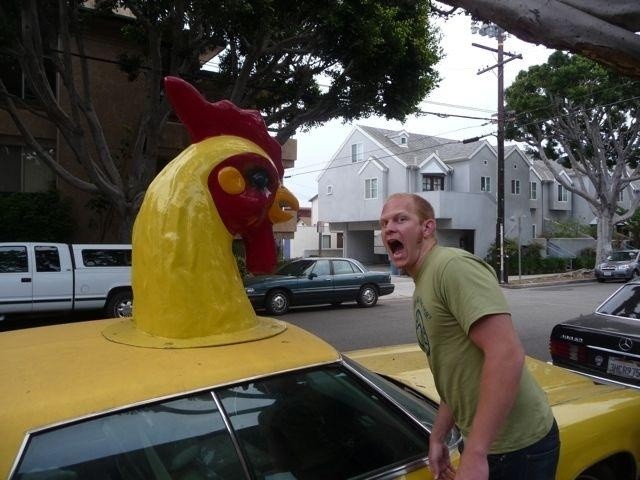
[377,192,560,480]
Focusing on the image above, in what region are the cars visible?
[244,257,394,316]
[594,250,640,283]
[549,277,640,383]
[0,74,640,480]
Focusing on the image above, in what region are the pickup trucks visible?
[0,242,133,318]
